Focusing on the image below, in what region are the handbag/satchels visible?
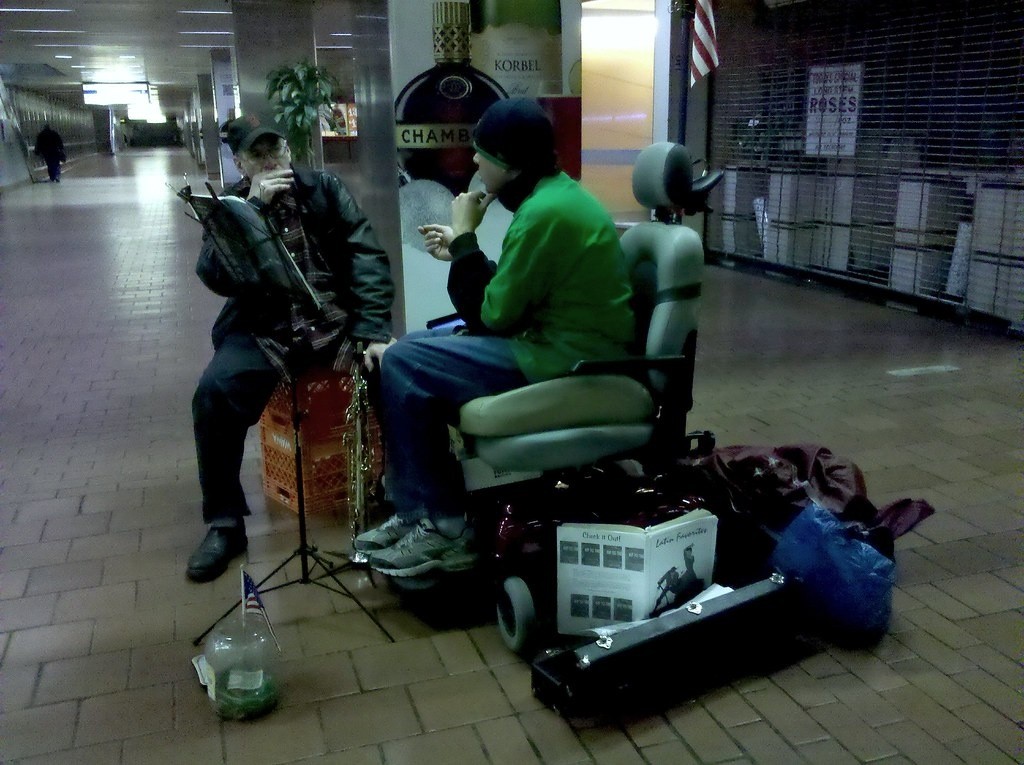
[768,503,894,633]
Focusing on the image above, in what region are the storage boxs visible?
[259,366,382,516]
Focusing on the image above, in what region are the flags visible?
[690,0,720,88]
[242,570,281,653]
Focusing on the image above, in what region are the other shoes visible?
[55,177,60,182]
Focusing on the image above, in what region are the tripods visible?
[176,181,396,645]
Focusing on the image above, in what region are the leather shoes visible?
[186,519,248,582]
[376,473,396,512]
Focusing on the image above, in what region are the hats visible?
[227,115,286,156]
[472,97,557,171]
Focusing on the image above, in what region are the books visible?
[557,507,719,637]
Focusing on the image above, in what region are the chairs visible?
[386,140,703,654]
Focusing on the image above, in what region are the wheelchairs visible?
[367,142,727,665]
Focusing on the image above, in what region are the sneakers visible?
[353,515,417,554]
[368,517,481,577]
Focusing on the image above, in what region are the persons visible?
[184,113,398,584]
[33,123,67,182]
[353,97,636,578]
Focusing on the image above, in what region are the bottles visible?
[394,0,508,194]
[206,613,279,721]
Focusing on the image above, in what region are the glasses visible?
[240,140,287,163]
